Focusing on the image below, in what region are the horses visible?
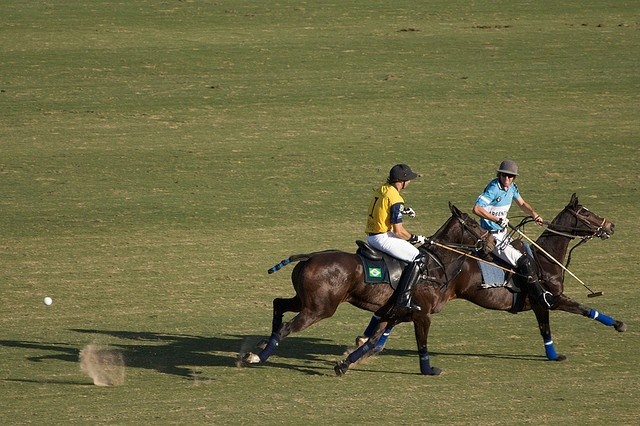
[242,202,496,364]
[334,193,627,377]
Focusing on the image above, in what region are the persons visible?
[364,164,428,312]
[472,160,563,310]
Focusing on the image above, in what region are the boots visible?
[394,261,422,312]
[516,266,559,309]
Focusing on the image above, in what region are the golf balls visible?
[43,297,52,306]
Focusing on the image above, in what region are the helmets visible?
[496,159,519,175]
[390,164,417,182]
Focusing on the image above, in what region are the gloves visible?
[534,215,543,226]
[401,207,416,218]
[406,234,426,243]
[495,218,510,227]
